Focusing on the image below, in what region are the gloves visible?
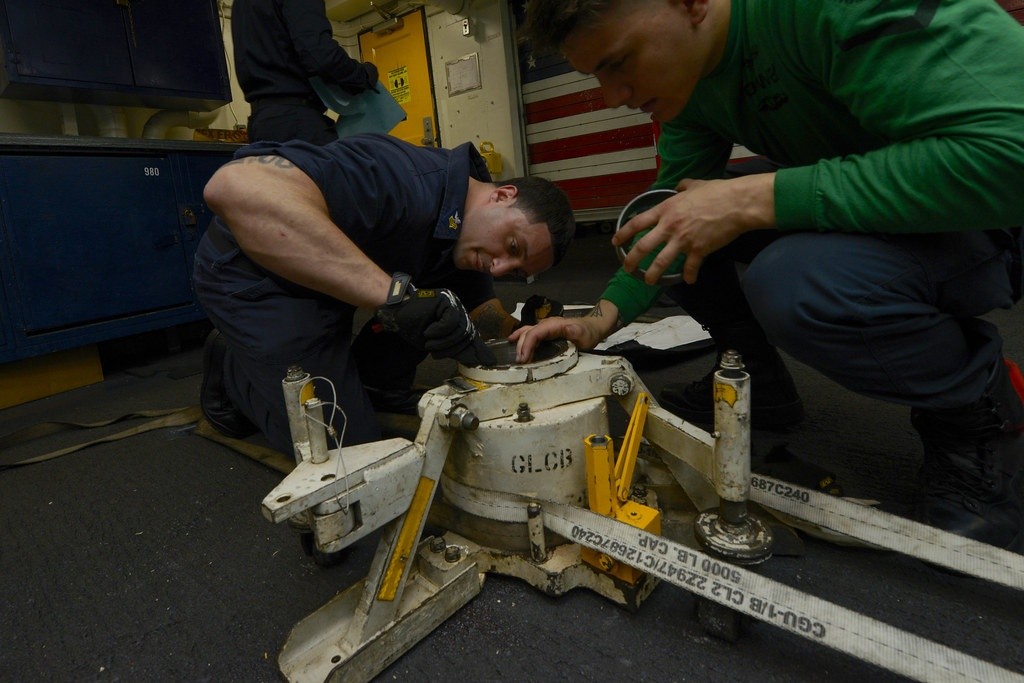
[375,270,499,370]
[511,294,564,332]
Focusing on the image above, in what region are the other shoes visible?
[361,383,427,415]
[199,329,257,439]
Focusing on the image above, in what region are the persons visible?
[189,132,577,455]
[507,1,1024,574]
[230,0,380,146]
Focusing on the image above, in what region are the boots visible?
[899,351,1024,563]
[658,307,806,431]
